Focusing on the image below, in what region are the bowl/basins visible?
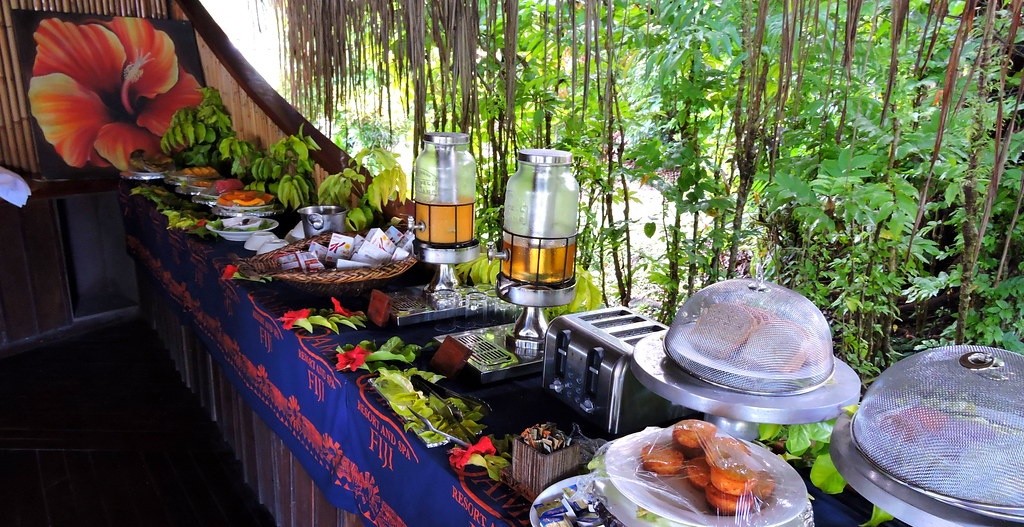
[206,216,279,241]
[291,221,305,238]
[244,232,279,251]
[256,239,289,255]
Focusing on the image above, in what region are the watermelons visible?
[209,179,243,196]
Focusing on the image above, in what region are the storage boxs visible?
[513,433,580,495]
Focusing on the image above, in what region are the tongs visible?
[406,393,479,449]
[410,374,492,422]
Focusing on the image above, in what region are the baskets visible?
[229,227,417,302]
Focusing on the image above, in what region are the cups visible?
[432,284,524,332]
[502,150,579,287]
[415,132,476,243]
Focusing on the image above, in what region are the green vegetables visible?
[374,366,487,442]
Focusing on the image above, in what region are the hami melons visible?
[217,191,274,206]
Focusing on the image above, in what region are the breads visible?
[688,302,816,373]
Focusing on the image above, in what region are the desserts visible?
[642,419,775,514]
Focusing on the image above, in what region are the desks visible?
[117,168,908,527]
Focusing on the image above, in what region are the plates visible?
[216,201,272,211]
[201,192,221,199]
[169,170,192,182]
[186,183,207,190]
[530,474,589,527]
[128,167,169,175]
[606,428,807,527]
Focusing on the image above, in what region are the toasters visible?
[540,306,701,434]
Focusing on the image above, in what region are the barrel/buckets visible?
[296,205,350,238]
